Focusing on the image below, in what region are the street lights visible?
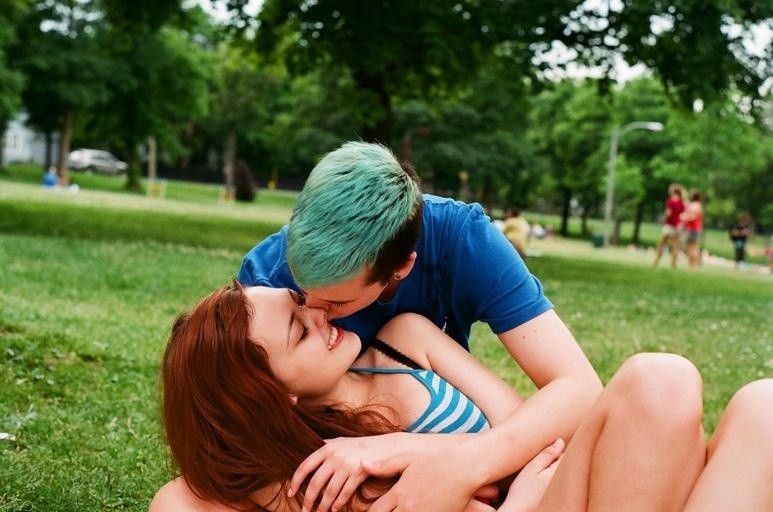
[601,120,666,250]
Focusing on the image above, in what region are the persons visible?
[145,138,607,512]
[42,165,57,188]
[500,205,530,259]
[727,211,752,271]
[650,185,686,269]
[677,192,703,267]
[158,282,773,511]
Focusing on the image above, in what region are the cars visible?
[66,147,128,178]
